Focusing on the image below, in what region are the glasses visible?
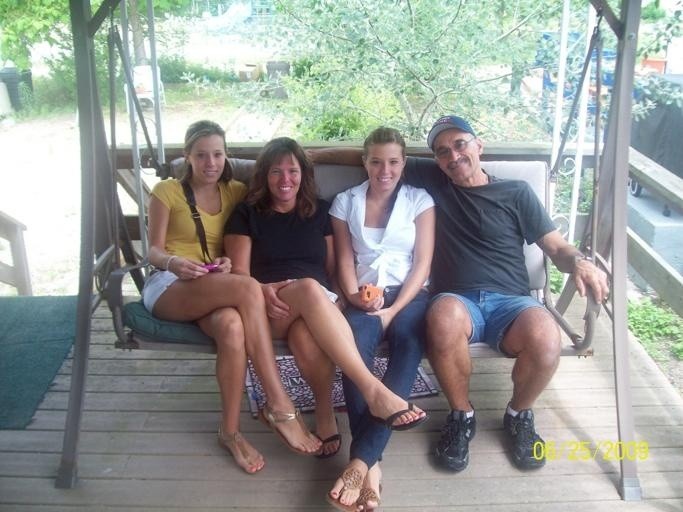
[432,135,475,159]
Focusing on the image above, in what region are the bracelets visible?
[166,256,179,271]
[568,255,592,266]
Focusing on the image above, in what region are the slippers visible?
[353,482,383,512]
[323,464,365,512]
[312,414,342,459]
[364,398,431,432]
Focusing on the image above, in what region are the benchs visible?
[123,162,596,357]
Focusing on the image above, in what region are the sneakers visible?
[433,400,477,474]
[502,400,547,469]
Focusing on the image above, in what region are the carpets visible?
[0,292,95,433]
[242,355,444,422]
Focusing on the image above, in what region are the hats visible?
[426,115,477,154]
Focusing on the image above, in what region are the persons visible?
[137,119,323,474]
[325,128,436,512]
[224,137,429,459]
[302,115,611,472]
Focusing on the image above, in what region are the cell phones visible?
[361,284,384,302]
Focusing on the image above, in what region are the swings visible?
[104,3,604,355]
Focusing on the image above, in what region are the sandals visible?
[217,423,264,474]
[258,400,324,456]
